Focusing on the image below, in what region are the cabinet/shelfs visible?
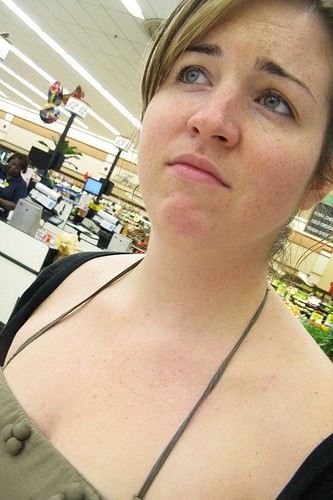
[44,169,151,234]
[0,139,29,166]
[267,261,333,330]
[0,207,133,336]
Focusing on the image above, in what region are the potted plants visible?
[38,133,83,172]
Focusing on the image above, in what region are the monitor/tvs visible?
[29,147,65,171]
[83,178,114,196]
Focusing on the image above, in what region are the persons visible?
[0,0,333,500]
[0,153,29,220]
